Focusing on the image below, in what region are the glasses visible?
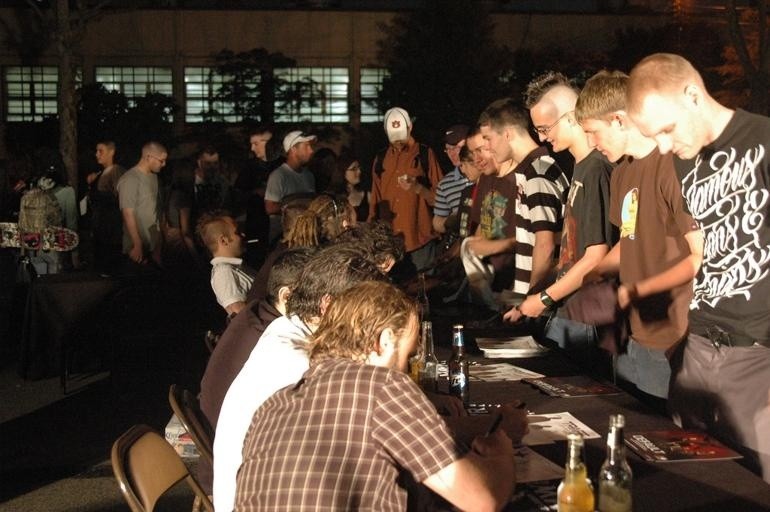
[153,155,168,164]
[347,166,362,171]
[444,146,462,153]
[533,112,575,136]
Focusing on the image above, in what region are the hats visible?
[384,108,412,143]
[443,124,473,146]
[283,130,317,153]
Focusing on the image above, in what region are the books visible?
[474,334,550,360]
[625,430,743,462]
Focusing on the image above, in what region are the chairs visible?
[205,311,240,356]
[167,383,217,464]
[111,423,213,512]
[279,192,318,222]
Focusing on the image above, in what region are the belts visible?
[703,327,756,348]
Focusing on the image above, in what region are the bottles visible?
[448,324,469,401]
[417,321,438,392]
[415,272,430,321]
[598,414,633,512]
[557,434,594,512]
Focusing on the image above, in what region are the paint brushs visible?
[485,400,529,434]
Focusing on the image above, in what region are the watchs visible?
[540,290,559,309]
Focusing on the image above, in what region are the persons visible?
[91,141,128,215]
[433,125,468,257]
[200,248,314,439]
[213,244,386,511]
[120,141,167,264]
[466,125,517,292]
[503,74,620,382]
[179,146,230,261]
[327,152,372,222]
[576,71,704,415]
[334,222,406,272]
[627,54,770,484]
[436,143,481,278]
[243,193,357,304]
[245,130,281,234]
[265,130,318,241]
[282,199,314,236]
[194,210,255,318]
[367,108,443,270]
[477,99,570,333]
[228,284,530,512]
